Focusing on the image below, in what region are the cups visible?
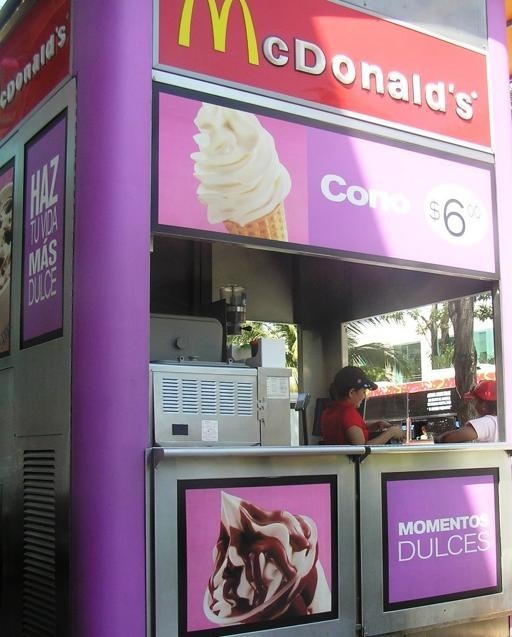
[203,515,333,627]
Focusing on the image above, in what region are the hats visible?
[463,380,497,401]
[334,367,378,390]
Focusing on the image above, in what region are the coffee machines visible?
[218,283,250,367]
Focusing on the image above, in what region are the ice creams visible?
[203,491,332,626]
[190,102,293,242]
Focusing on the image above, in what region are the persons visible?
[433,379,498,443]
[419,425,428,439]
[319,365,406,445]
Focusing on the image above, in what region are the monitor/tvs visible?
[312,396,367,436]
